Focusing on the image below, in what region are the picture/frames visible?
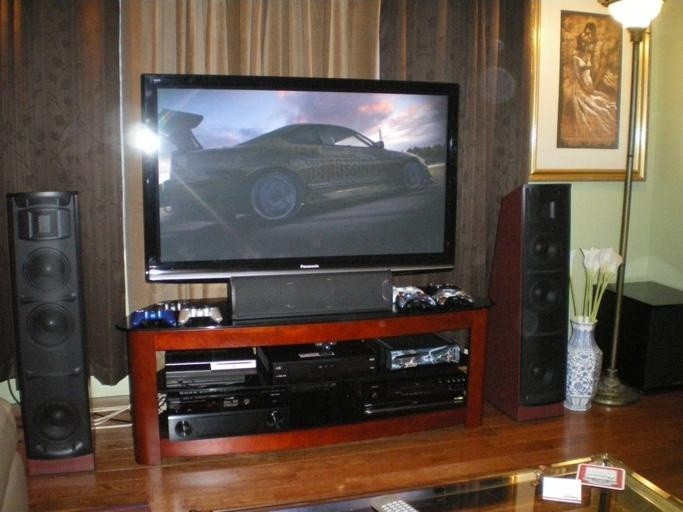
[520,2,655,186]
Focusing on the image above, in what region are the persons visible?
[585,22,617,98]
[567,33,617,143]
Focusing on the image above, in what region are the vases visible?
[563,317,609,417]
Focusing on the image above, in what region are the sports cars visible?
[158,108,431,225]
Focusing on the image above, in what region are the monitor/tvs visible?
[140,73,460,284]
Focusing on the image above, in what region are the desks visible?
[116,295,493,467]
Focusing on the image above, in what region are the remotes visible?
[372,496,418,512]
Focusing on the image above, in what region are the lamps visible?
[597,0,670,50]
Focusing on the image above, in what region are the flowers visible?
[564,249,621,326]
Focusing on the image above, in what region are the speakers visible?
[228,271,393,321]
[7,191,94,476]
[485,183,571,422]
[593,281,683,395]
